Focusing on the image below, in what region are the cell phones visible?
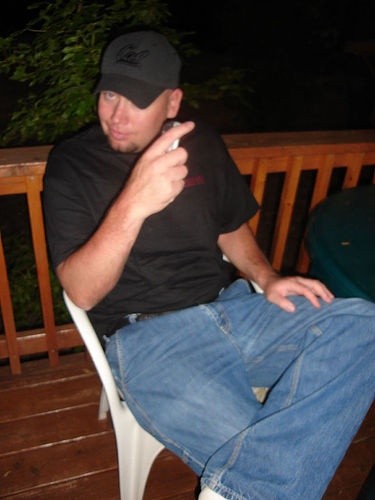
[160,121,181,153]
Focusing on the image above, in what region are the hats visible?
[91,32,180,109]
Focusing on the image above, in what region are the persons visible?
[42,31,375,500]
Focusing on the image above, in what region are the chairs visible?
[64,294,164,500]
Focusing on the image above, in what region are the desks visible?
[301,184,375,500]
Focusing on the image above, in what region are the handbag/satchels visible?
[284,185,375,303]
